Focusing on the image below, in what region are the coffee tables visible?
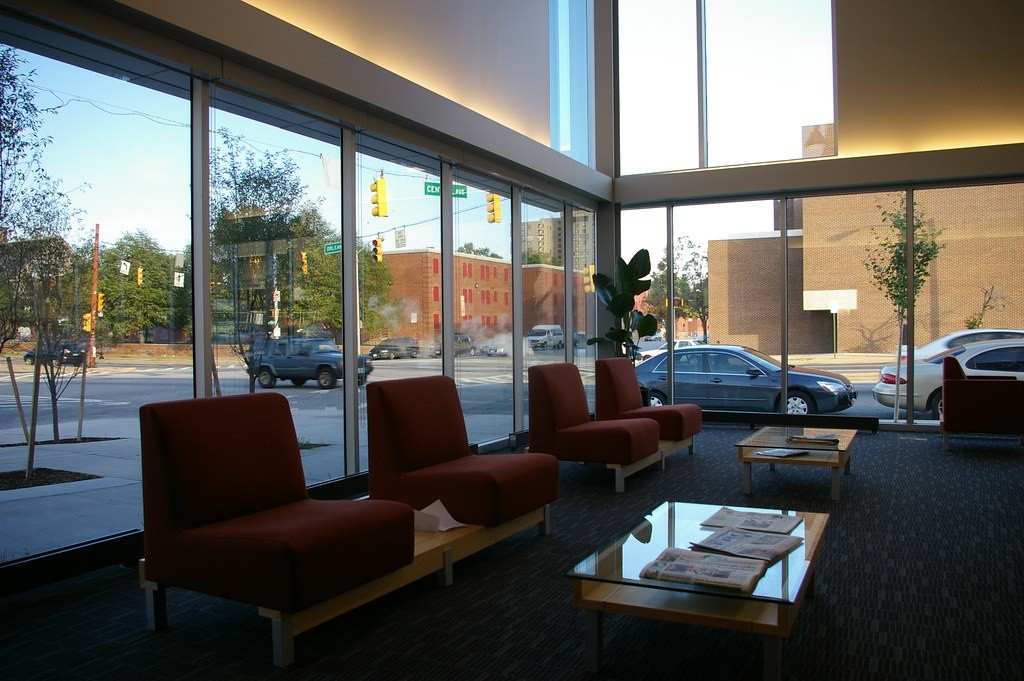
[733,425,859,502]
[566,499,833,681]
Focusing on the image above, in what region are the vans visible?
[369,336,419,359]
[528,325,564,350]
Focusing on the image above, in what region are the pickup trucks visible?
[247,338,374,390]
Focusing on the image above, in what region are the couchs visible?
[940,354,1024,451]
[525,364,660,498]
[591,357,703,454]
[137,391,417,670]
[365,372,563,537]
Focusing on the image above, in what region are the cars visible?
[212,319,266,344]
[873,337,1024,420]
[634,344,857,415]
[640,340,720,362]
[24,341,84,366]
[480,340,509,357]
[903,327,1024,363]
[644,333,662,341]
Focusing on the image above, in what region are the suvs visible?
[439,333,496,356]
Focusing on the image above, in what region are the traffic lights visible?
[98,293,105,312]
[373,238,382,262]
[138,267,144,286]
[299,252,308,274]
